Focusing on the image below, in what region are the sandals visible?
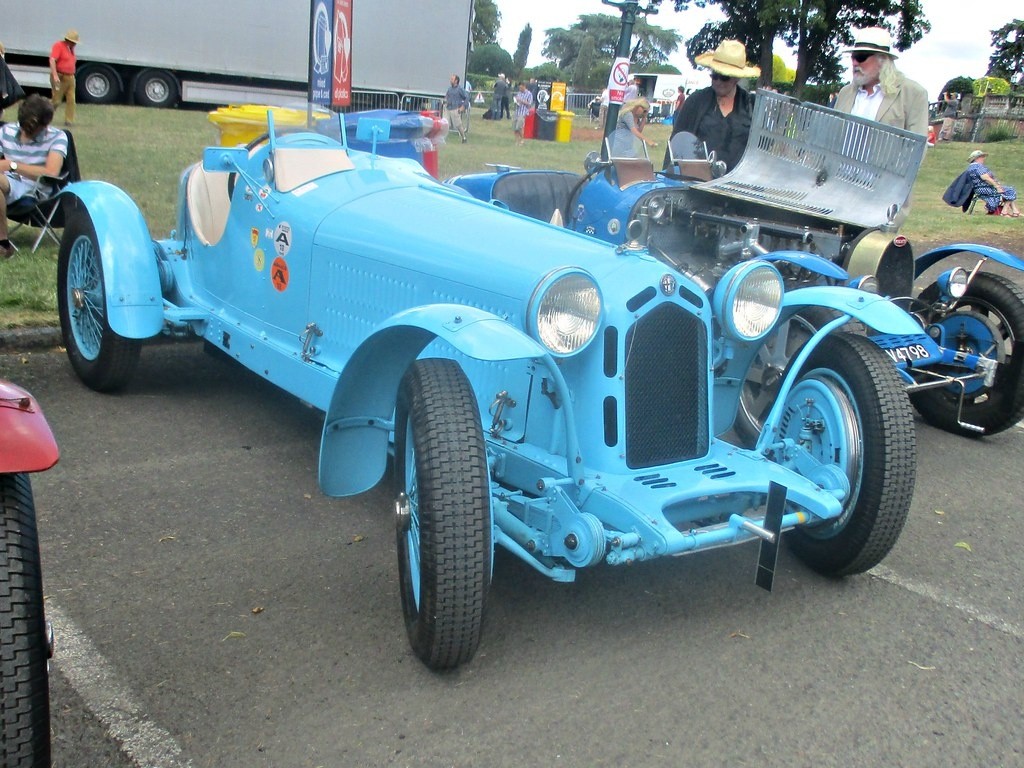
[1012,212,1024,217]
[1000,212,1013,218]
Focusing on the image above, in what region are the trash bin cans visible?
[555,111,575,143]
[524,108,535,138]
[535,80,552,110]
[536,109,558,141]
[206,104,331,148]
[315,109,433,167]
[550,82,566,111]
[420,110,449,179]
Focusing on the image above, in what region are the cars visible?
[52,98,931,673]
[450,100,1024,436]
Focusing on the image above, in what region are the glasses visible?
[710,71,735,82]
[640,105,648,114]
[851,52,877,63]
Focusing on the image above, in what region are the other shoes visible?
[461,138,466,143]
[65,121,71,127]
[0,243,16,259]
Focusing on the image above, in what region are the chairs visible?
[965,169,1003,215]
[647,103,676,123]
[0,120,81,253]
[494,173,589,227]
[588,102,605,122]
[186,146,262,247]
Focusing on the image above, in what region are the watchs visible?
[9,160,17,172]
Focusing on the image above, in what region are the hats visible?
[63,30,80,44]
[841,27,899,61]
[497,73,505,78]
[967,150,988,164]
[694,40,761,78]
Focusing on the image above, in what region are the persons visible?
[50,30,80,127]
[672,86,685,127]
[511,82,533,146]
[926,126,936,148]
[938,91,958,140]
[827,90,838,108]
[755,83,793,133]
[612,97,656,158]
[588,85,610,129]
[0,95,68,258]
[662,39,778,179]
[491,73,512,119]
[623,80,638,103]
[966,150,1024,218]
[441,74,472,143]
[0,41,25,116]
[834,27,929,139]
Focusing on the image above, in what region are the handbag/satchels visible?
[0,56,26,111]
